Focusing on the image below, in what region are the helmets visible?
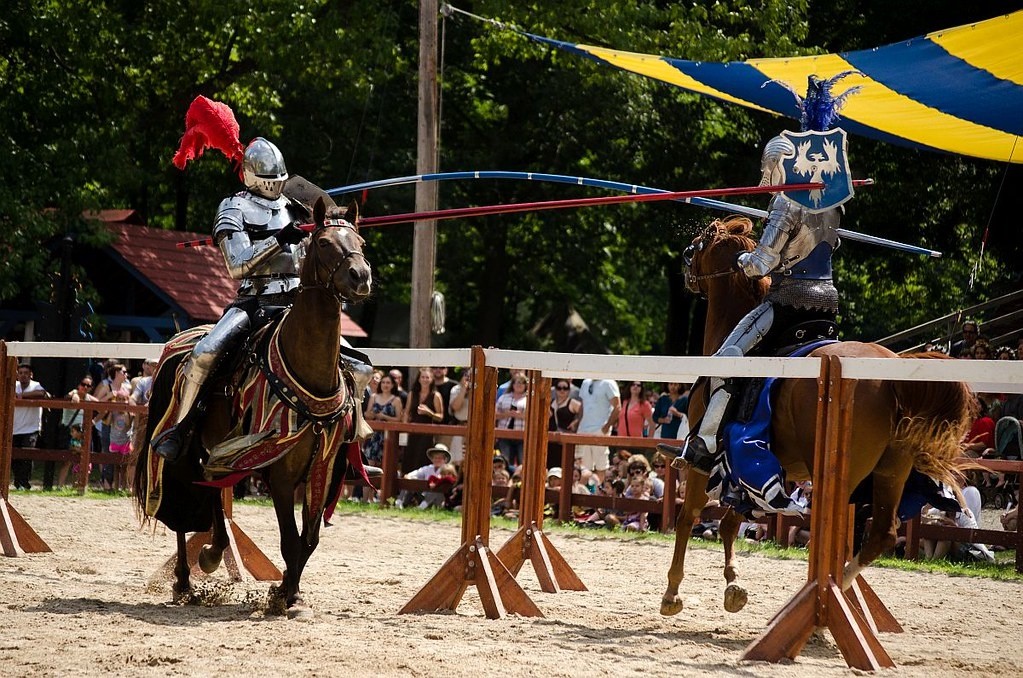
[758,135,797,194]
[241,137,288,199]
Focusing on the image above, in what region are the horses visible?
[124,195,374,622]
[659,217,999,615]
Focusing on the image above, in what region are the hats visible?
[426,444,452,464]
[547,467,563,479]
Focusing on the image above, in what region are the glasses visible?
[630,469,642,477]
[653,465,666,468]
[120,370,126,374]
[555,386,569,391]
[80,382,93,389]
[632,384,642,387]
[650,401,656,404]
[588,384,593,395]
[963,330,974,334]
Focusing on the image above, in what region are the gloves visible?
[274,221,310,251]
[731,250,750,272]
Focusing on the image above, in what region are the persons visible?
[42,359,158,491]
[151,137,353,475]
[491,368,693,533]
[656,137,847,475]
[344,367,470,511]
[854,320,1023,562]
[12,363,45,490]
[692,476,813,548]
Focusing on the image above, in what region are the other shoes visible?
[57,485,62,491]
[155,438,182,462]
[656,436,716,475]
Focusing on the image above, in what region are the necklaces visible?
[556,397,567,405]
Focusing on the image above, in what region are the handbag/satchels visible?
[56,423,73,447]
